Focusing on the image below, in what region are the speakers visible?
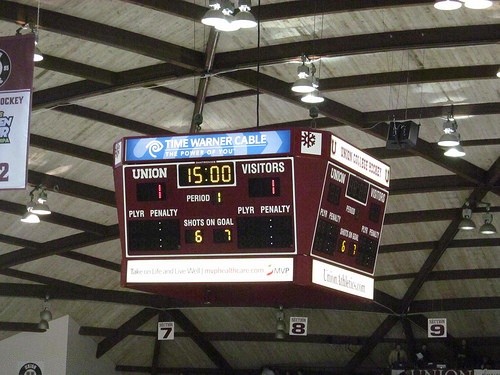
[386,120,419,149]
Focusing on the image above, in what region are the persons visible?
[388,339,495,370]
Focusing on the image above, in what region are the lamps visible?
[433,0,492,10]
[29,17,43,62]
[438,111,466,158]
[39,294,52,329]
[458,198,497,235]
[291,52,324,103]
[201,0,257,31]
[20,183,51,223]
[275,305,286,338]
